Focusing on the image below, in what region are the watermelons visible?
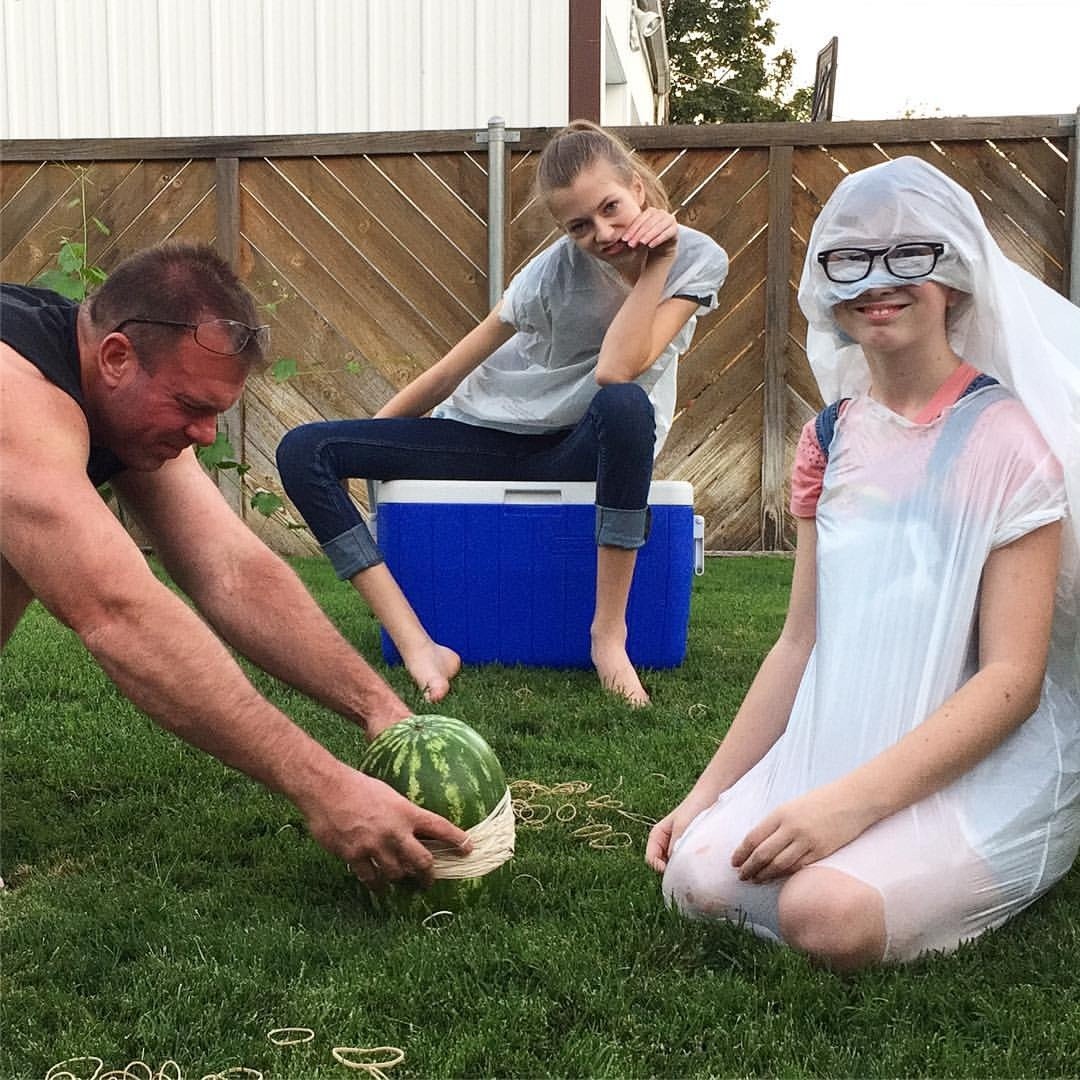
[360,714,506,839]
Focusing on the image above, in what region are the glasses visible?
[112,316,275,358]
[816,243,948,285]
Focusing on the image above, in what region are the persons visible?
[645,153,1079,976]
[0,239,476,893]
[275,117,731,708]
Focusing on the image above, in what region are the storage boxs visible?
[376,479,704,669]
